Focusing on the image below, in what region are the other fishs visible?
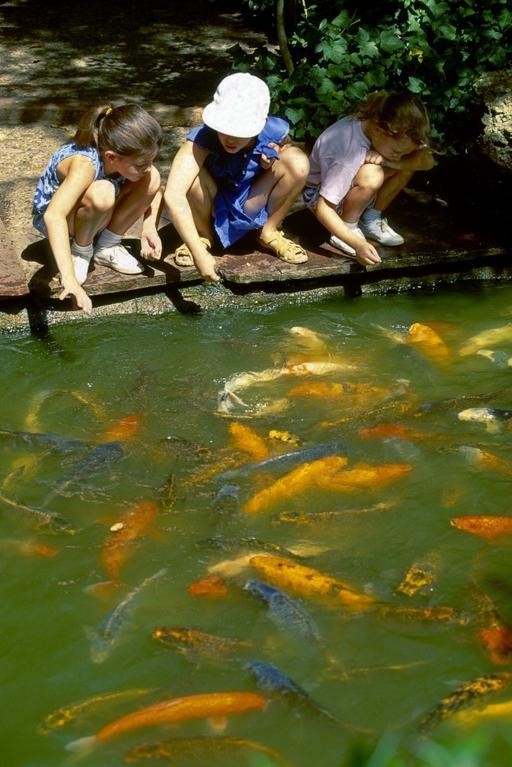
[1,314,512,766]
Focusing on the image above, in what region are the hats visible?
[203,71,271,138]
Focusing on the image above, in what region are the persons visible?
[298,86,439,268]
[159,69,312,286]
[28,99,167,318]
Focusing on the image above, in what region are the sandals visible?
[257,229,308,264]
[174,234,211,266]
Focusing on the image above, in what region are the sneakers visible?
[94,244,145,275]
[360,217,405,247]
[330,226,369,256]
[71,243,94,286]
